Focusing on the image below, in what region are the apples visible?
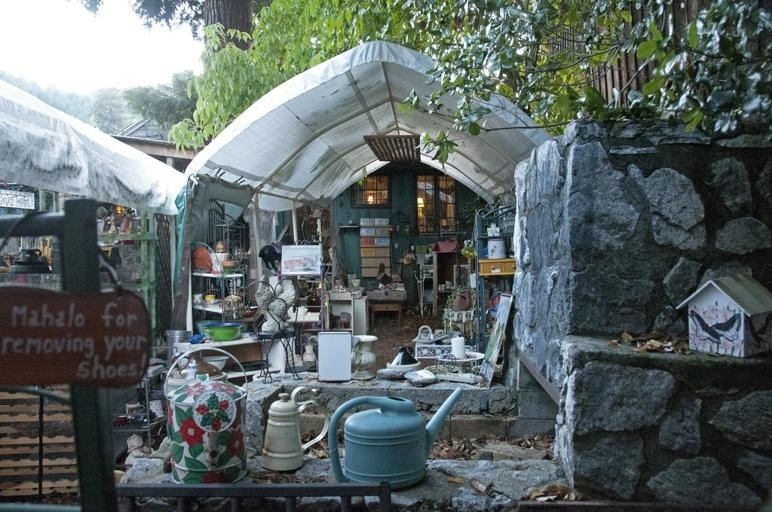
[451,335,466,359]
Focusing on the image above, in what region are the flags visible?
[351,333,379,381]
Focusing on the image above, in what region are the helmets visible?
[190,242,215,274]
[232,360,268,372]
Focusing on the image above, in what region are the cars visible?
[222,260,239,273]
[438,284,442,290]
[446,281,452,289]
[441,284,446,290]
[352,279,361,291]
[210,252,233,274]
[205,294,215,301]
[487,238,508,259]
[125,400,146,417]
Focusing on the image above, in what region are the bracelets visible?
[195,320,220,333]
[203,356,229,372]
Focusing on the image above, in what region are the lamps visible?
[245,278,305,385]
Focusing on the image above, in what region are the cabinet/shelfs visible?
[351,333,379,382]
[303,345,315,361]
[307,291,322,312]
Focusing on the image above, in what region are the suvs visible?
[162,346,249,485]
[164,329,191,357]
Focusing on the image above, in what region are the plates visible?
[471,184,516,355]
[106,345,179,473]
[173,332,287,388]
[302,206,336,291]
[354,295,371,335]
[190,272,245,323]
[325,294,355,335]
[432,250,458,318]
[300,292,323,332]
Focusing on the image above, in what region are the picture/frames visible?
[286,310,320,358]
[414,263,433,317]
[367,285,407,325]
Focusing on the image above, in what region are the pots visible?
[206,322,242,341]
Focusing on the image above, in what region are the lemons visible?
[259,386,330,470]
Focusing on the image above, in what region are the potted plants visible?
[280,245,322,276]
[478,292,514,387]
